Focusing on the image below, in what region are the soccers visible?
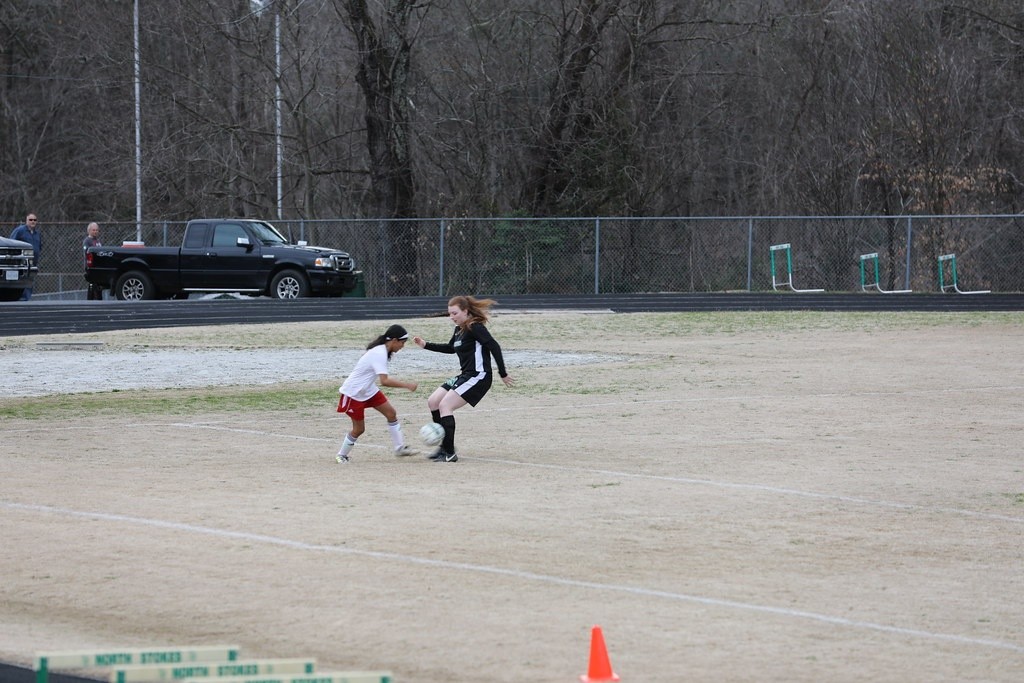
[421,423,445,446]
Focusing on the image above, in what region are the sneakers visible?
[394,445,420,457]
[335,454,349,464]
[428,447,458,462]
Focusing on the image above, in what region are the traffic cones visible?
[579,626,621,682]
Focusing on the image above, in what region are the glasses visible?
[28,219,37,221]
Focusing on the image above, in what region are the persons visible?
[333,323,420,464]
[82,221,103,300]
[411,295,517,463]
[9,213,43,301]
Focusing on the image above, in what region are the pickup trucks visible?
[84,219,359,299]
